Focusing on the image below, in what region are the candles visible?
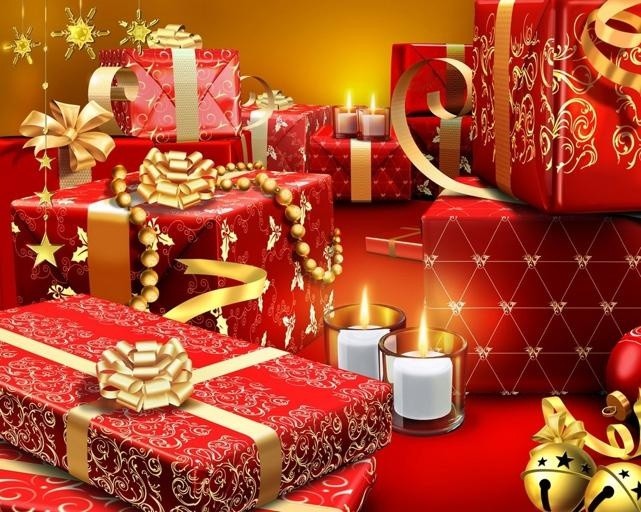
[337,109,386,136]
[336,321,455,422]
